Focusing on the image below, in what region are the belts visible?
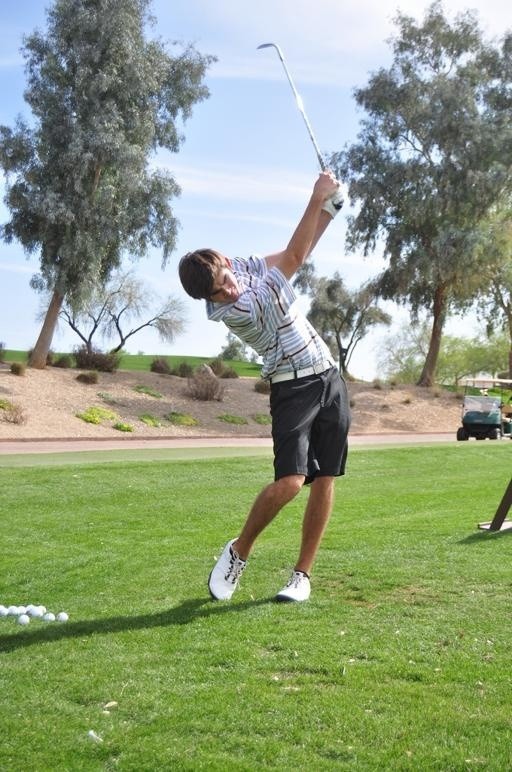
[270,357,336,384]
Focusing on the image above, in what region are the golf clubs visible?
[258,43,342,211]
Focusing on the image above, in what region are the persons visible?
[175,163,352,608]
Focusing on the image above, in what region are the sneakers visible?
[208,537,247,602]
[276,571,311,601]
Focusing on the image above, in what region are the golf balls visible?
[0,603,69,626]
